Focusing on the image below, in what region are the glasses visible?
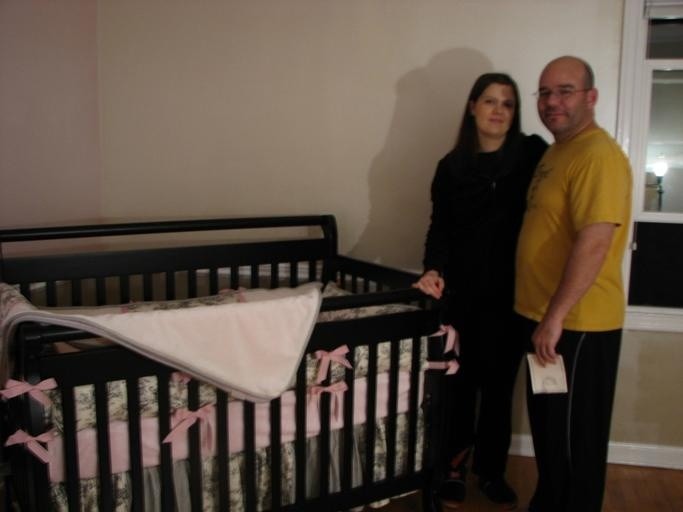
[532,85,594,102]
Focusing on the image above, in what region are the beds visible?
[1,214,449,512]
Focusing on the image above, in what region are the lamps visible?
[653,151,669,212]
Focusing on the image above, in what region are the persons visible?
[514,56,632,512]
[411,73,549,511]
